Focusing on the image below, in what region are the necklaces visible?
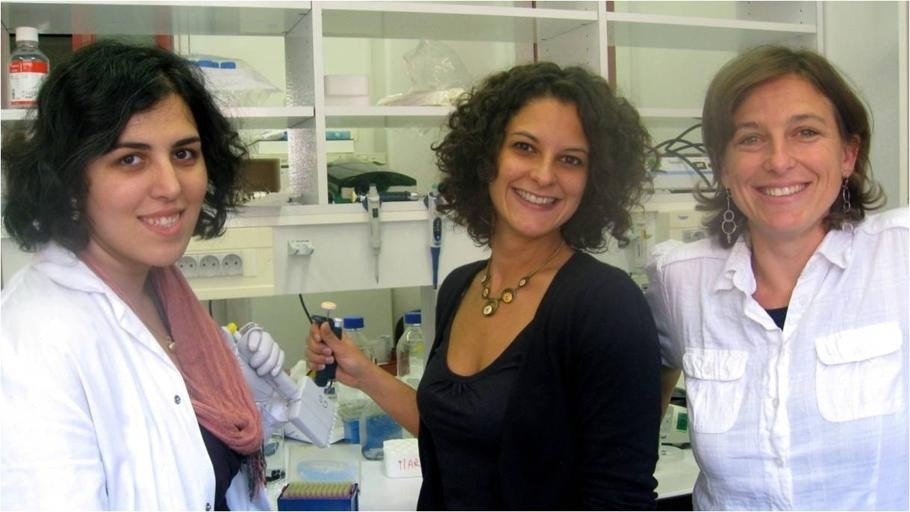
[78,248,177,353]
[480,240,566,317]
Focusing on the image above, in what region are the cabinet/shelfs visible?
[0,3,827,304]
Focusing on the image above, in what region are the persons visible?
[1,41,273,511]
[647,44,908,511]
[305,62,660,511]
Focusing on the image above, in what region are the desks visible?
[258,354,716,512]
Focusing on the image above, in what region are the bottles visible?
[8,25,52,109]
[314,301,425,459]
